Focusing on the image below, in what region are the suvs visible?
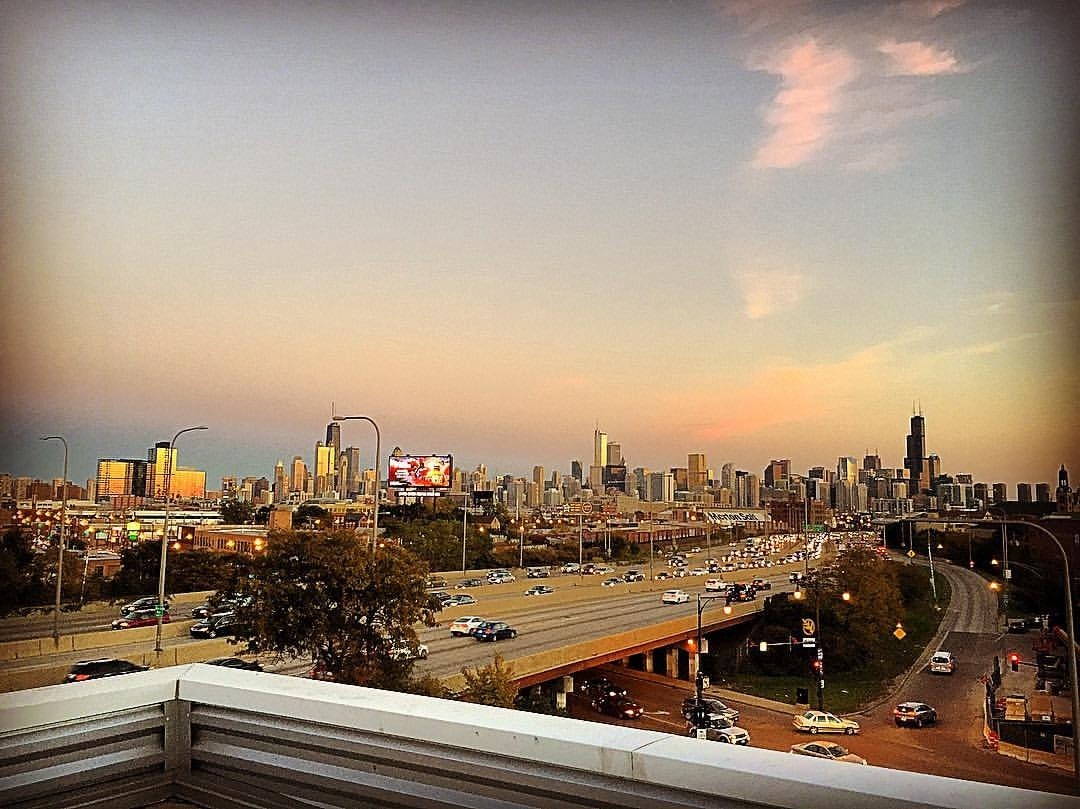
[68,658,150,686]
[894,702,937,728]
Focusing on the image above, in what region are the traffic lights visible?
[1011,654,1019,672]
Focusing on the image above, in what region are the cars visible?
[385,530,890,667]
[930,651,957,673]
[112,593,279,673]
[583,677,869,765]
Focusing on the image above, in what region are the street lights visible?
[988,559,1050,628]
[332,414,381,579]
[39,432,69,638]
[154,423,210,654]
